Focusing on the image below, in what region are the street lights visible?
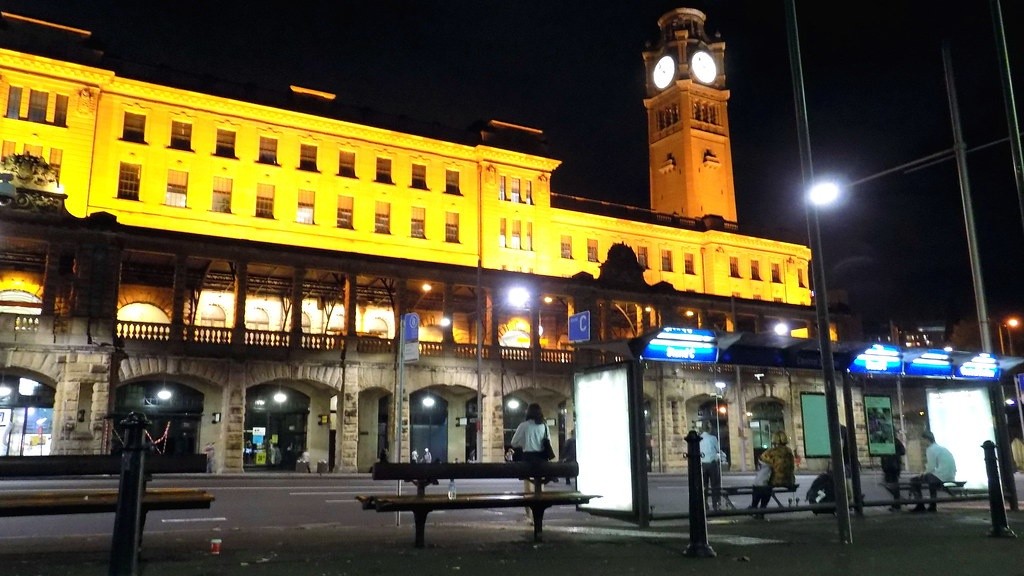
[810,45,1019,516]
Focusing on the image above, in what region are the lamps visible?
[273,380,286,403]
[158,375,172,400]
[423,387,434,407]
[508,392,519,409]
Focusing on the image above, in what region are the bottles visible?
[447,478,456,500]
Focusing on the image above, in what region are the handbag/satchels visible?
[543,441,555,460]
[753,458,772,488]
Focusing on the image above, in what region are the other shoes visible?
[926,507,938,512]
[911,506,926,512]
[887,508,901,514]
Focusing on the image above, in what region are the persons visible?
[469,449,476,462]
[828,424,852,502]
[379,446,389,462]
[424,447,431,464]
[881,427,906,512]
[748,432,796,518]
[510,403,550,522]
[505,448,514,463]
[699,420,721,511]
[1010,431,1024,501]
[561,429,577,484]
[412,449,417,463]
[908,431,956,512]
[717,450,726,462]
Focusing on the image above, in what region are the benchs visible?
[0,453,217,565]
[355,461,603,549]
[878,481,968,515]
[704,483,801,523]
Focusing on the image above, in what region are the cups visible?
[210,539,222,554]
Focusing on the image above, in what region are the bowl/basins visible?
[953,481,967,487]
